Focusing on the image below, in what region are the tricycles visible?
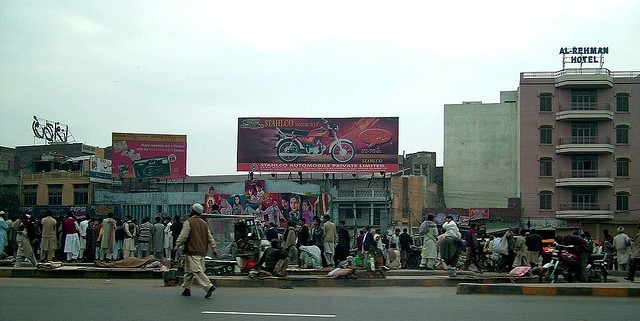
[200,214,270,276]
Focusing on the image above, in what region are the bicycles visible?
[399,247,419,269]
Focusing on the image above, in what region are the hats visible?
[193,203,203,213]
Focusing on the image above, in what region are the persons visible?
[144,216,154,256]
[593,240,600,254]
[64,212,80,263]
[248,181,265,202]
[211,204,220,214]
[356,230,364,251]
[624,220,640,281]
[173,203,219,298]
[280,196,291,221]
[207,200,213,213]
[602,229,614,252]
[573,232,577,236]
[266,223,278,241]
[418,215,439,270]
[6,214,13,231]
[122,215,137,259]
[217,195,232,214]
[321,215,338,266]
[284,221,296,243]
[86,221,95,262]
[602,235,615,270]
[243,201,255,214]
[333,220,350,268]
[256,204,263,213]
[310,216,319,232]
[525,231,530,238]
[435,216,459,267]
[99,213,117,262]
[0,211,9,257]
[295,217,309,268]
[552,235,563,246]
[9,216,23,257]
[257,237,288,276]
[613,226,632,271]
[133,219,139,234]
[313,187,332,219]
[136,219,151,258]
[55,216,64,261]
[463,224,483,273]
[399,228,413,269]
[498,227,516,274]
[94,218,103,260]
[525,228,543,277]
[91,217,97,227]
[382,234,389,249]
[152,216,166,259]
[164,216,173,258]
[23,211,43,259]
[141,219,146,224]
[113,221,125,261]
[286,222,298,265]
[482,232,502,272]
[512,229,531,267]
[373,228,383,250]
[39,210,57,263]
[170,215,184,262]
[97,226,104,242]
[289,194,302,221]
[262,198,280,225]
[583,231,593,245]
[361,226,373,254]
[300,198,315,224]
[390,228,401,251]
[232,195,243,214]
[310,218,328,268]
[78,215,91,259]
[13,223,38,268]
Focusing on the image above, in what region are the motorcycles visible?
[539,242,610,283]
[275,118,355,163]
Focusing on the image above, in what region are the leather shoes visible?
[205,287,215,298]
[624,277,634,281]
[182,290,190,296]
